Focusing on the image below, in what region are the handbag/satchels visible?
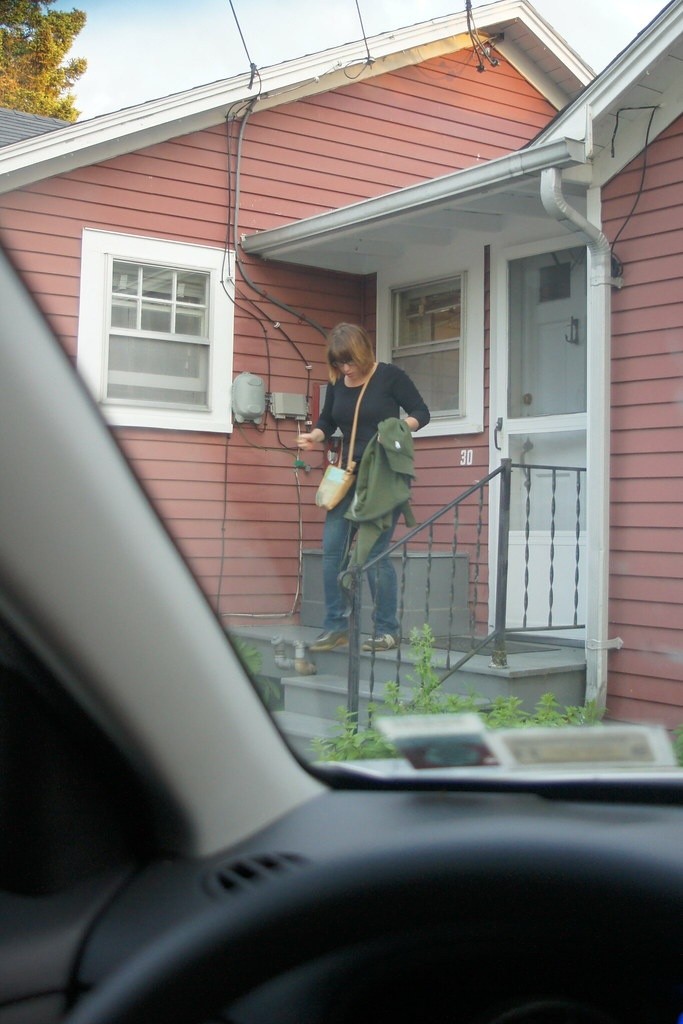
[314,464,356,512]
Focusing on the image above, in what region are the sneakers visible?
[309,630,350,652]
[362,632,399,651]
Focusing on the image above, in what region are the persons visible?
[292,323,431,651]
